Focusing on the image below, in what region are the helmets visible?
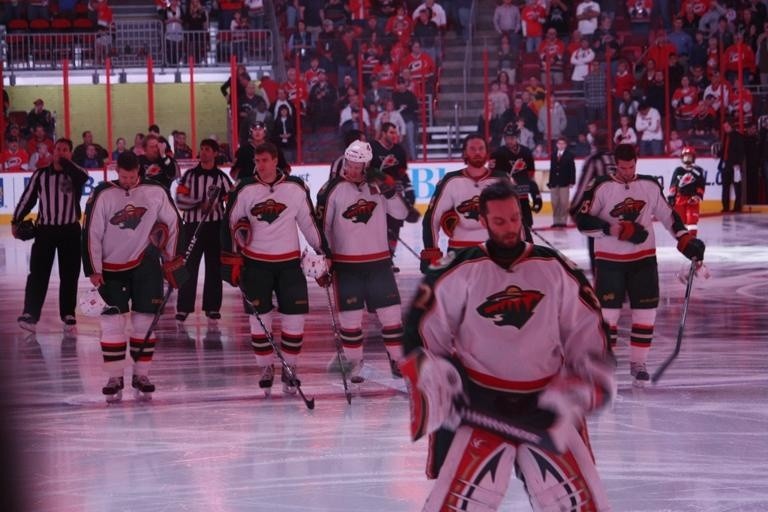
[504,124,520,138]
[248,121,267,136]
[344,140,373,182]
[17,218,35,241]
[681,146,695,164]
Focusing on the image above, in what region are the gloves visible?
[420,249,442,271]
[366,169,394,190]
[149,222,168,249]
[233,220,252,249]
[531,194,542,213]
[312,255,331,286]
[677,235,705,261]
[688,195,700,206]
[163,256,184,288]
[220,252,242,287]
[618,220,648,243]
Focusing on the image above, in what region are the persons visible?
[479,0,767,158]
[1,1,113,65]
[11,121,419,403]
[155,1,469,163]
[396,182,619,511]
[1,89,191,169]
[423,123,748,386]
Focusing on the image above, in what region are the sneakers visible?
[176,311,188,320]
[349,365,364,382]
[132,374,154,392]
[205,310,221,318]
[18,313,37,324]
[281,364,300,386]
[631,361,649,380]
[259,364,274,387]
[103,376,124,394]
[61,312,76,325]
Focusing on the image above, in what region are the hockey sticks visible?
[237,279,315,409]
[650,257,696,382]
[523,221,593,272]
[325,284,352,406]
[130,186,220,363]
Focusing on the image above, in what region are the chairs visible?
[507,0,679,134]
